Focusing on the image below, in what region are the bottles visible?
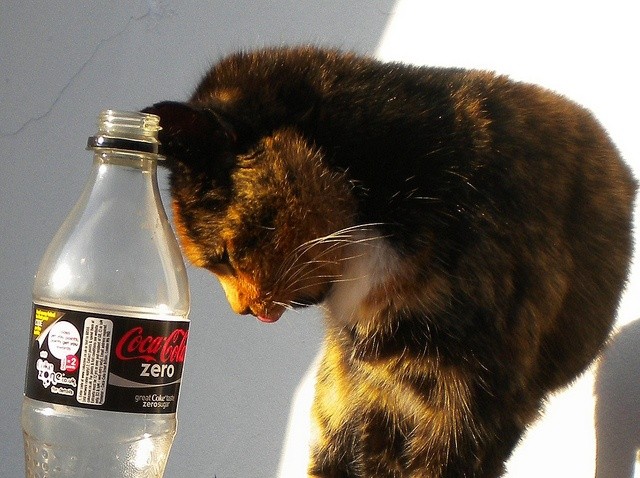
[22,108,190,478]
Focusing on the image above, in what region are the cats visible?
[137,46,639,478]
